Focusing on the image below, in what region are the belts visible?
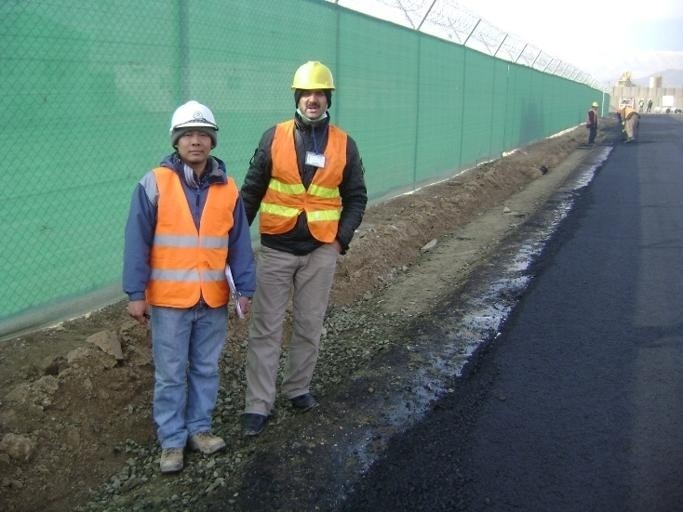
[189,296,212,311]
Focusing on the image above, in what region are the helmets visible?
[291,61,336,90]
[592,102,598,107]
[170,100,218,131]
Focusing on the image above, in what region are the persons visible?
[620,107,640,143]
[586,101,599,145]
[645,98,652,112]
[122,97,256,475]
[237,58,367,437]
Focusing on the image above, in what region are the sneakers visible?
[190,432,226,455]
[245,414,266,436]
[160,448,184,473]
[292,394,321,414]
